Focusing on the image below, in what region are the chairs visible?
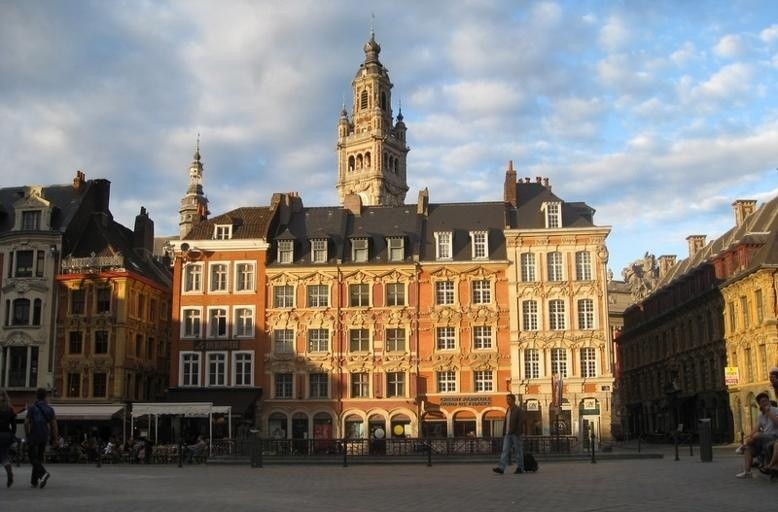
[7,437,231,468]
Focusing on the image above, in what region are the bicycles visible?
[214,438,346,454]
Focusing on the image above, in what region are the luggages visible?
[523,453,539,473]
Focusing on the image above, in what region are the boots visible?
[5,463,14,487]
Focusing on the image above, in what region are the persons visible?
[22,387,58,487]
[50,426,207,466]
[492,394,525,474]
[0,388,17,487]
[735,368,778,479]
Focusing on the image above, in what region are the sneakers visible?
[39,472,50,488]
[30,483,37,488]
[514,468,522,473]
[493,468,504,473]
